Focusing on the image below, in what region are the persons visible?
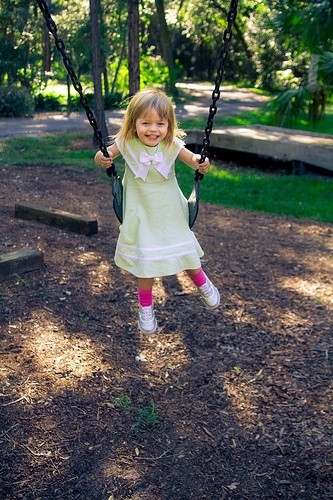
[94,89,220,334]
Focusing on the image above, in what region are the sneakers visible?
[197,270,220,310]
[138,302,157,334]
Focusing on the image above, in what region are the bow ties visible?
[139,151,164,163]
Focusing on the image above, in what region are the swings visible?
[35,0,238,228]
